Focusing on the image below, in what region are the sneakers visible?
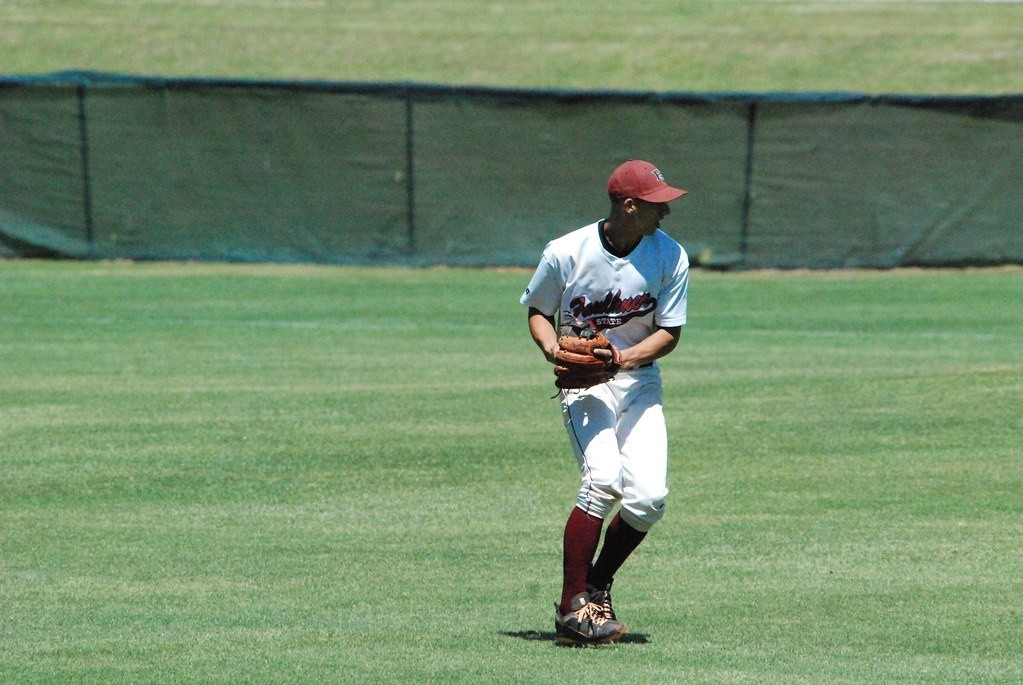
[586,565,616,622]
[553,591,625,645]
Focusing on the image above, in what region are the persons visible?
[519,159,689,645]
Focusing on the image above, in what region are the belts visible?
[639,361,653,369]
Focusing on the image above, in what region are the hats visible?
[608,160,687,204]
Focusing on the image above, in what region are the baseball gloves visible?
[553,315,622,389]
